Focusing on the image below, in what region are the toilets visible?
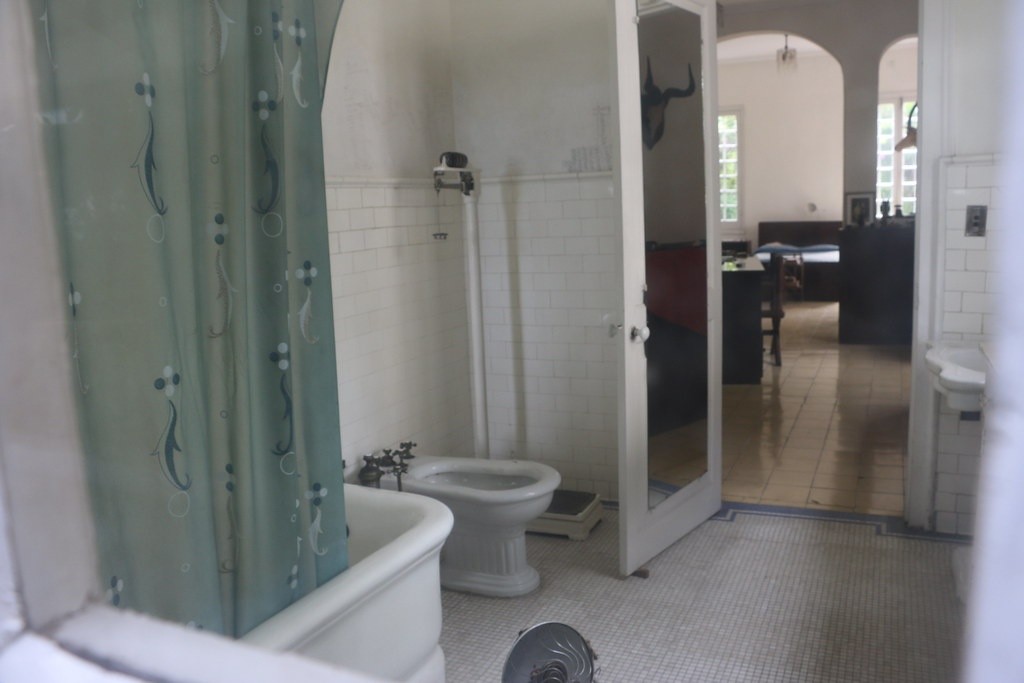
[369,452,566,601]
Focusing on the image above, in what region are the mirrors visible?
[638,1,710,514]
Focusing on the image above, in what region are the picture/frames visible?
[844,191,876,229]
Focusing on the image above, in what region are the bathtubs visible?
[232,474,448,682]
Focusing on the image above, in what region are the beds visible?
[749,241,841,301]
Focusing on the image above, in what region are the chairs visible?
[759,252,786,367]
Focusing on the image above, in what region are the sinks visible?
[926,336,986,419]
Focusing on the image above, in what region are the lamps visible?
[772,32,800,84]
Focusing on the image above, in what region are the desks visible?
[721,249,775,384]
